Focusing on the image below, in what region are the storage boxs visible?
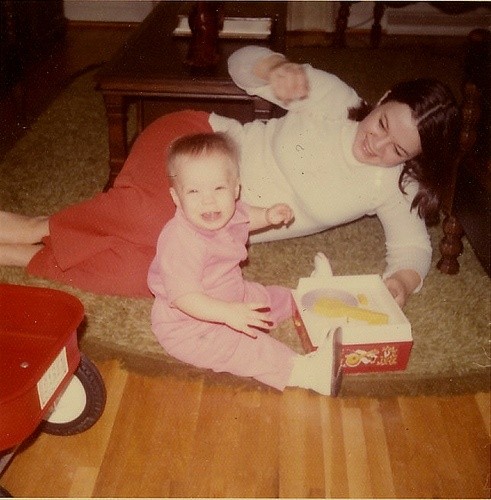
[289,273,415,377]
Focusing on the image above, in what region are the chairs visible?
[92,0,289,194]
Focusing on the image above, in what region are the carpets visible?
[0,62,491,398]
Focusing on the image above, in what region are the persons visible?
[0,45,465,314]
[145,126,345,400]
